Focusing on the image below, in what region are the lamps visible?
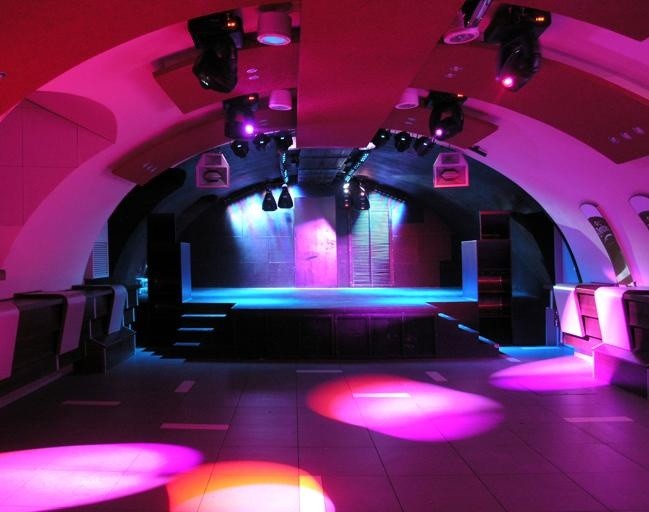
[397,87,423,114]
[338,176,371,212]
[231,131,294,158]
[256,12,293,47]
[260,184,294,213]
[444,12,479,46]
[431,151,472,190]
[373,127,432,157]
[267,91,294,111]
[194,150,231,191]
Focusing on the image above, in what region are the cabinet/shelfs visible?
[460,210,514,342]
